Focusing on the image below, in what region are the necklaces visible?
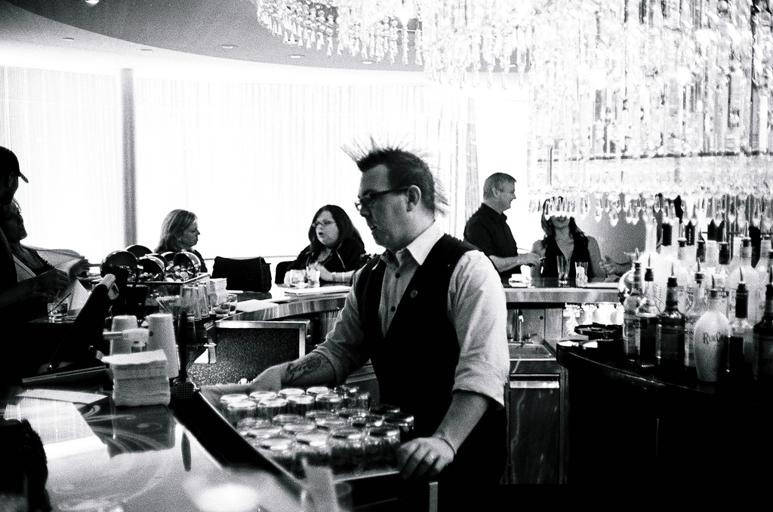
[560,236,570,245]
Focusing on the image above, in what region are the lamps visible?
[250,0,773,237]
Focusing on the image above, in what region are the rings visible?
[428,452,434,460]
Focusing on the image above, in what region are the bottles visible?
[617,217,772,387]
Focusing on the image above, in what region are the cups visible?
[301,265,322,291]
[289,266,305,293]
[555,253,571,287]
[104,315,142,365]
[572,260,591,287]
[146,312,182,380]
[530,255,547,286]
[175,264,238,327]
[46,302,69,322]
[217,383,418,471]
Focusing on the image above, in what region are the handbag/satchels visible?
[211,256,271,293]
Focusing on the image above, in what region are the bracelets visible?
[516,254,521,265]
[431,430,457,453]
[341,271,345,281]
[331,271,336,282]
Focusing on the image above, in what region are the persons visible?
[530,195,608,277]
[282,204,371,288]
[206,140,509,482]
[462,172,541,287]
[153,210,208,273]
[0,145,71,315]
[1,200,90,301]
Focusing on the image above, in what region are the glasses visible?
[355,186,404,211]
